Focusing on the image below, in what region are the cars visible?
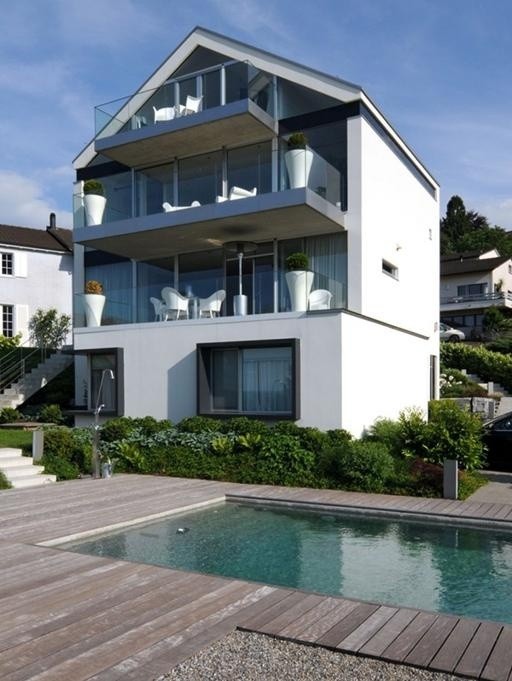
[469,410,512,474]
[439,321,468,344]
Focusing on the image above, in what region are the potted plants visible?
[285,132,314,190]
[83,180,107,226]
[81,281,105,327]
[284,253,314,311]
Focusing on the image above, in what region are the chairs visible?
[153,95,203,125]
[216,186,257,203]
[307,289,332,310]
[150,286,227,321]
